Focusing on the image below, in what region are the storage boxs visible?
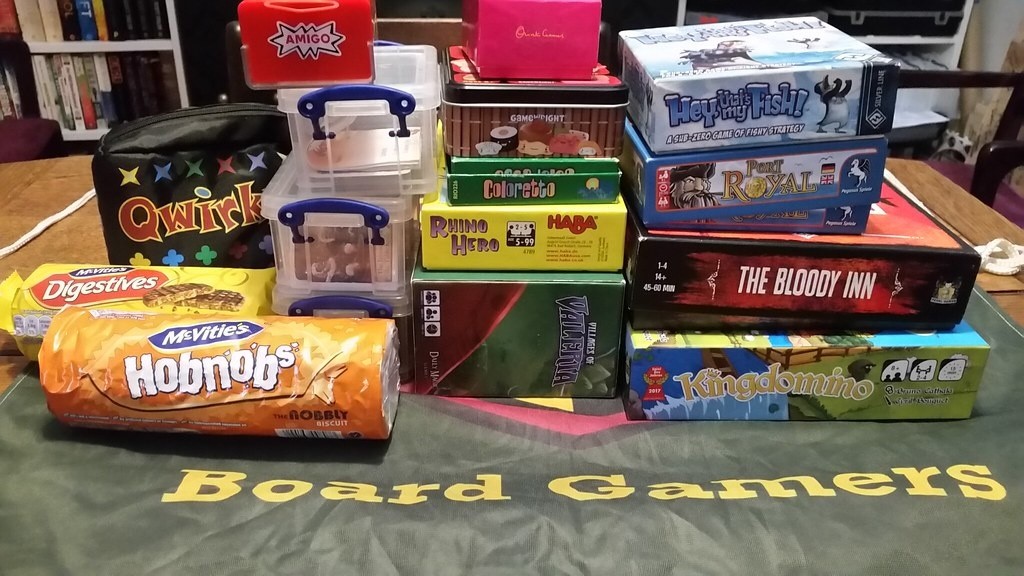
[436,49,630,162]
[269,283,415,387]
[617,15,901,161]
[418,177,630,273]
[273,39,446,195]
[408,236,629,400]
[443,154,622,207]
[260,145,428,306]
[622,320,991,423]
[620,116,889,241]
[620,170,984,336]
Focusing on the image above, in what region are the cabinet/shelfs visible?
[0,0,199,148]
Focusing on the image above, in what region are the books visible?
[0,0,182,132]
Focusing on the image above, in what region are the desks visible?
[4,148,1021,576]
